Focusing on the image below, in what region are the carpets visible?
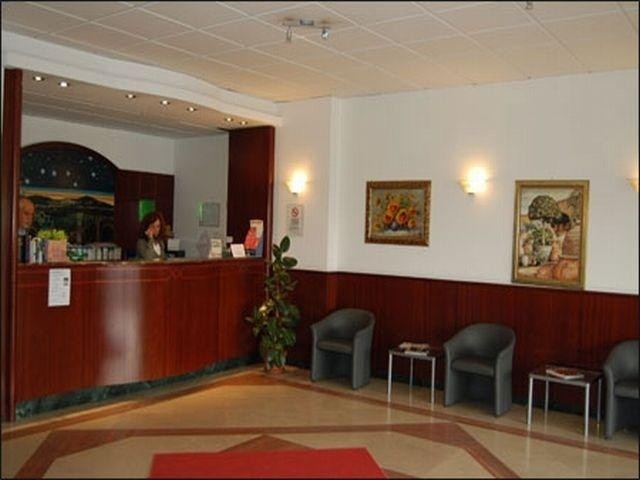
[150,445,388,479]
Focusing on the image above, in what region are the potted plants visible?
[245,235,300,373]
[527,194,562,263]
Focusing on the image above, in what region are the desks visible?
[525,365,604,436]
[387,346,440,402]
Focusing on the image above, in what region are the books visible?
[398,340,432,358]
[544,364,584,381]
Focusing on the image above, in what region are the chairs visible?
[309,307,376,391]
[444,323,515,417]
[604,340,640,443]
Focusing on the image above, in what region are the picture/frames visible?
[510,178,588,290]
[365,178,430,246]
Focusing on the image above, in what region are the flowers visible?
[372,191,420,232]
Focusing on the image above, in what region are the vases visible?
[389,221,400,231]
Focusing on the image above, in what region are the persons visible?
[134,210,166,261]
[18,195,36,261]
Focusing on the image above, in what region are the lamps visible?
[286,168,307,193]
[456,164,491,194]
[628,176,638,192]
